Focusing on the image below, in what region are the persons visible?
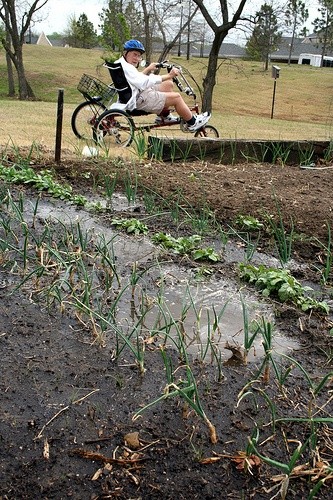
[113,39,212,133]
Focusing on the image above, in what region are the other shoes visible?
[188,111,211,131]
[154,113,179,123]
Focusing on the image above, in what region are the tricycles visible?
[71,59,219,148]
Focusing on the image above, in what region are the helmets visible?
[123,40,146,55]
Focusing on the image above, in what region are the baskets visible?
[77,72,115,106]
